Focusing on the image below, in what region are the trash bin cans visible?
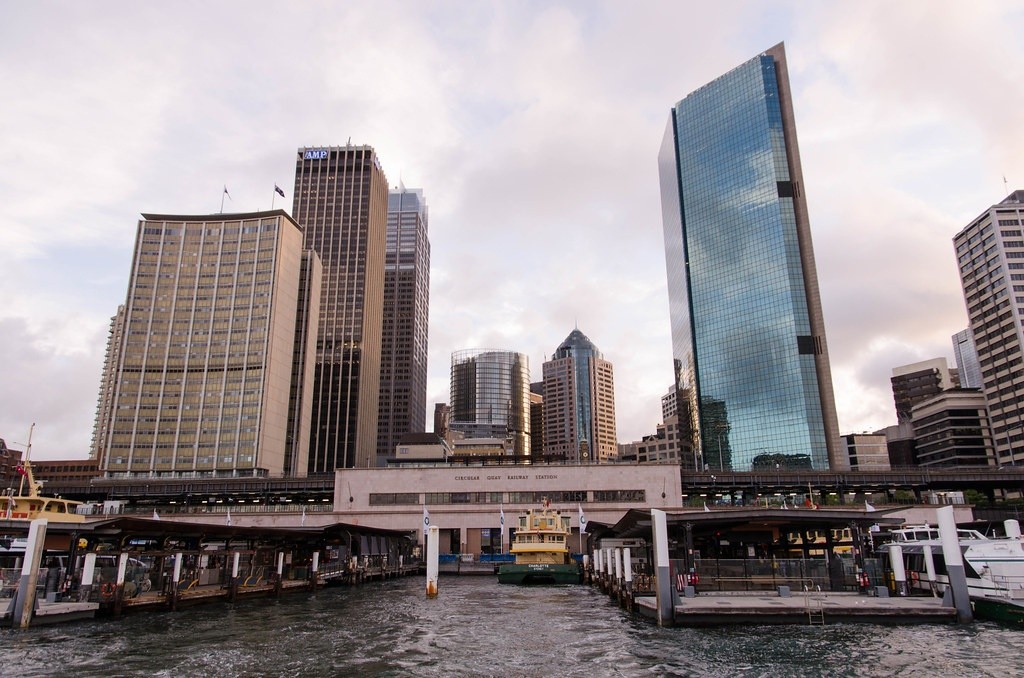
[296,566,308,581]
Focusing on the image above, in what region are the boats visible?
[874,517,1024,624]
[493,495,584,586]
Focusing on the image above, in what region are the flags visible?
[17,465,29,478]
[424,508,431,536]
[275,185,285,198]
[500,509,507,536]
[579,506,588,533]
[225,188,232,199]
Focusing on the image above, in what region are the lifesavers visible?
[905,570,917,584]
[101,580,116,597]
